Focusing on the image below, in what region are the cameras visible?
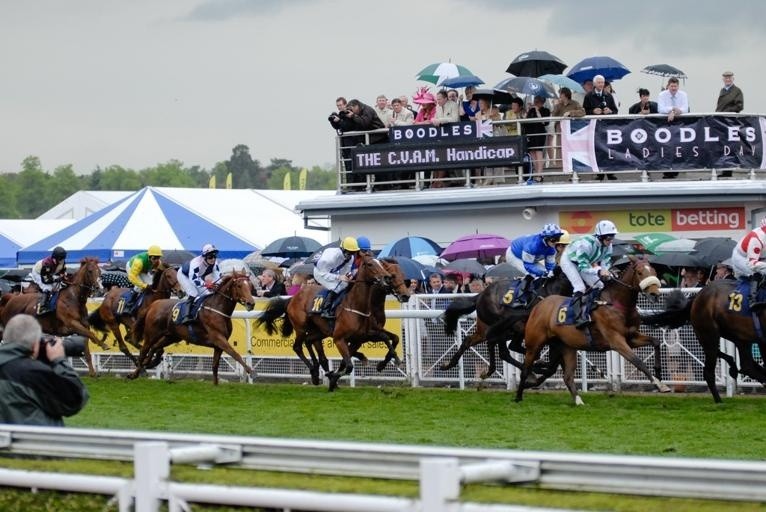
[329,112,337,122]
[599,102,606,110]
[40,333,85,363]
[339,109,352,118]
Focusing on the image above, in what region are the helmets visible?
[593,220,619,236]
[52,246,67,260]
[540,223,564,237]
[357,236,371,250]
[340,237,360,252]
[202,244,219,256]
[148,245,162,256]
[556,229,571,244]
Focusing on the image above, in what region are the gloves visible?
[203,279,214,289]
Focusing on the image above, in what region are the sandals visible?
[534,176,544,182]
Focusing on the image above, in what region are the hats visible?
[722,71,734,76]
[413,92,436,104]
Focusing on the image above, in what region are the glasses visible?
[549,236,560,243]
[360,250,370,253]
[605,235,614,241]
[152,256,160,260]
[346,251,356,255]
[207,254,217,259]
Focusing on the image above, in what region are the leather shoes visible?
[595,176,604,180]
[608,175,617,180]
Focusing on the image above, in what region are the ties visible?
[672,97,675,106]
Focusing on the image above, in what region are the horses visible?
[0,256,110,378]
[509,252,673,407]
[88,262,183,378]
[279,255,410,392]
[126,268,258,386]
[441,260,590,389]
[638,278,766,404]
[252,249,402,378]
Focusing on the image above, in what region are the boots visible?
[510,274,534,309]
[121,289,138,315]
[320,290,336,319]
[571,291,594,329]
[749,281,766,311]
[182,295,195,325]
[34,289,51,317]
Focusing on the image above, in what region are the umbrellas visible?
[506,48,568,77]
[640,63,689,87]
[567,56,631,83]
[415,56,474,91]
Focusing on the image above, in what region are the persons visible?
[0,313,91,428]
[327,68,691,190]
[0,221,765,329]
[714,71,745,178]
